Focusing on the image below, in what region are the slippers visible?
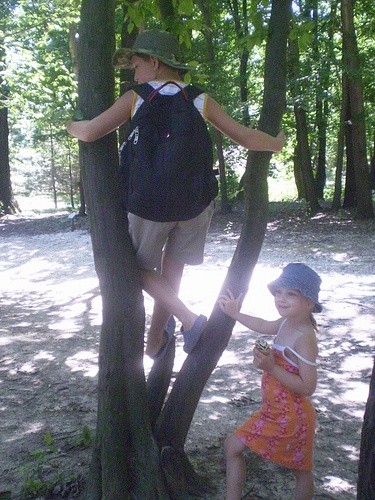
[180,314,207,355]
[147,315,176,362]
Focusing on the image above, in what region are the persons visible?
[215,261,323,500]
[67,28,287,363]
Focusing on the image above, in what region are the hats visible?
[111,30,199,71]
[267,262,322,313]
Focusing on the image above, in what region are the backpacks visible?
[117,81,219,223]
